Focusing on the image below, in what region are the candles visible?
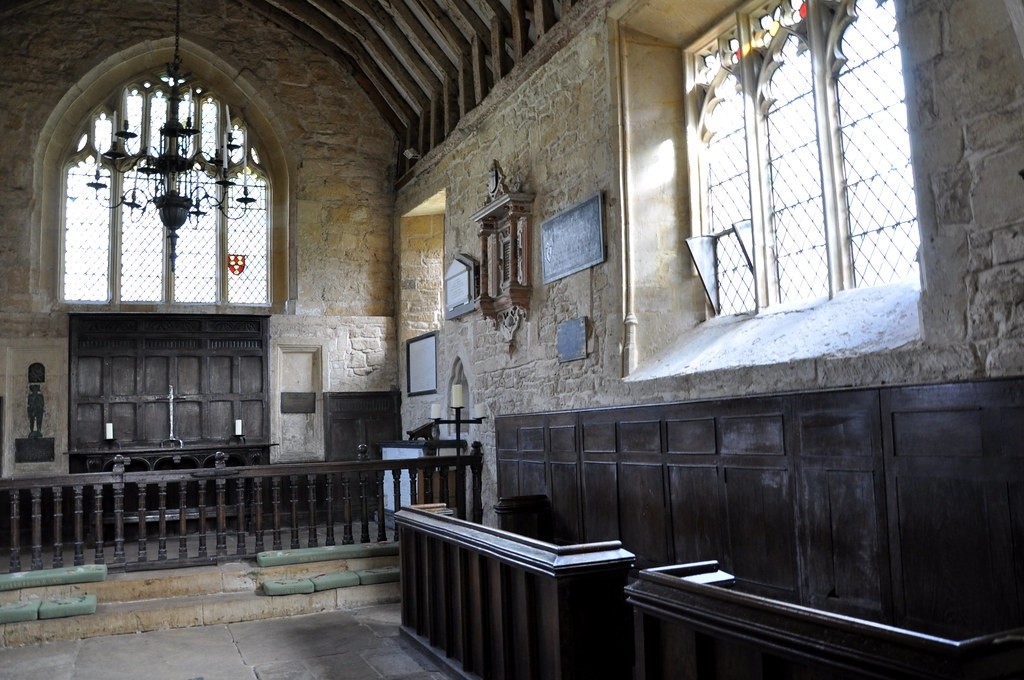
[223,133,228,168]
[235,419,242,435]
[124,87,128,120]
[430,403,441,419]
[452,384,462,407]
[96,142,101,170]
[188,88,192,117]
[243,155,248,186]
[226,104,232,133]
[105,423,113,439]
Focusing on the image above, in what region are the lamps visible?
[92,0,255,273]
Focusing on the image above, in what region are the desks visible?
[62,443,279,535]
